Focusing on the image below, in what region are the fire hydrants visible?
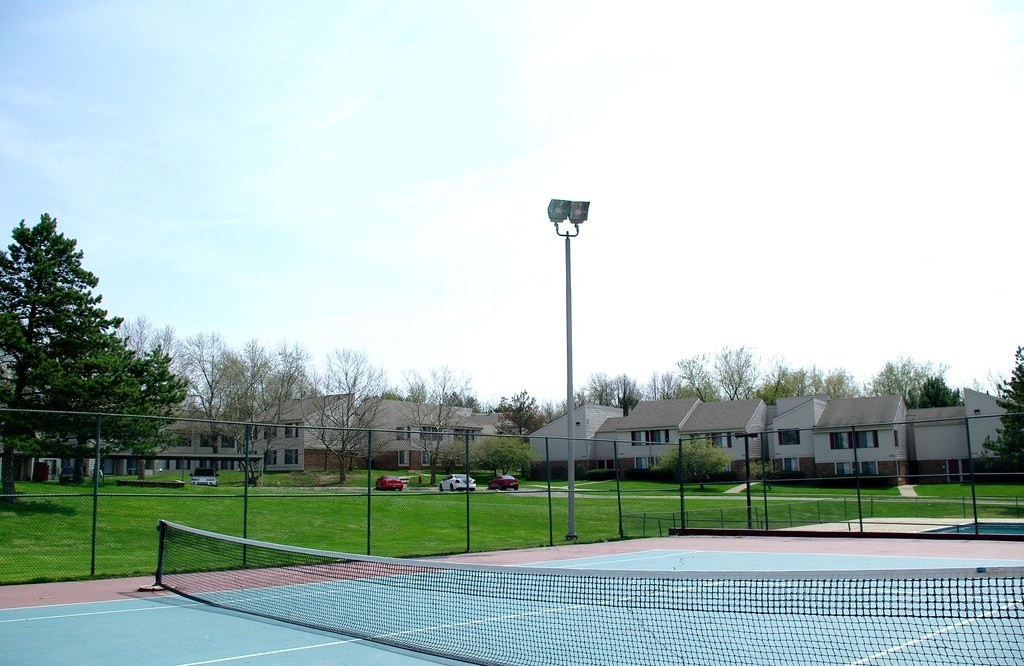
[418,476,422,484]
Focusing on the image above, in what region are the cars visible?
[488,474,518,491]
[189,468,220,486]
[376,475,403,491]
[439,474,476,492]
[58,467,84,484]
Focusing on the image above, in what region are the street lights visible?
[547,199,590,540]
